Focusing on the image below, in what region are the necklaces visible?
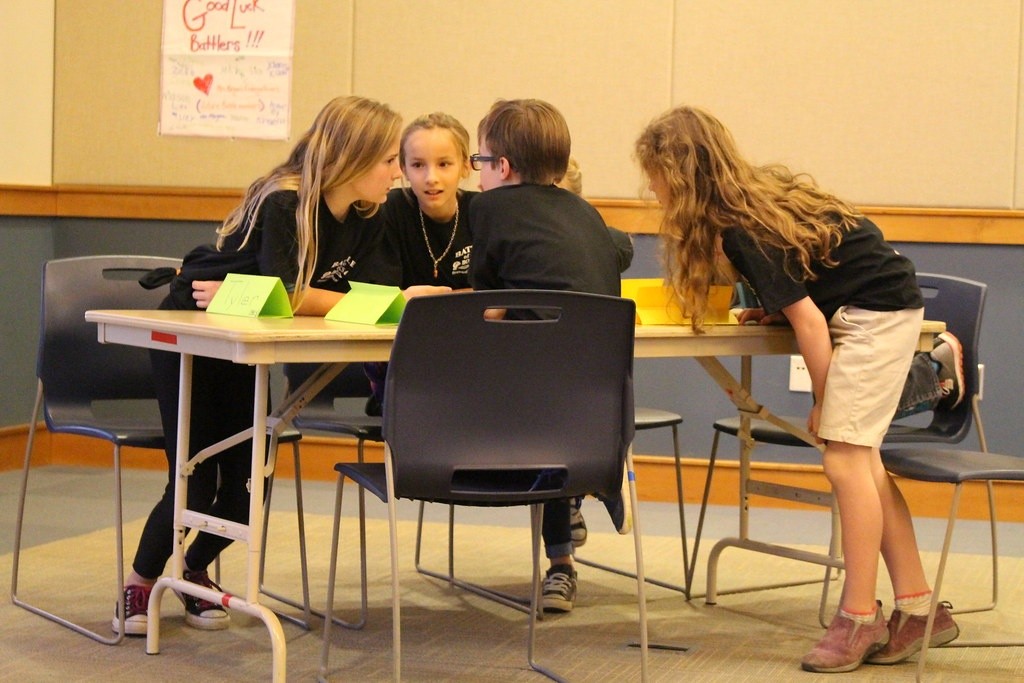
[419,197,460,278]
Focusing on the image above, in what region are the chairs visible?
[257,362,543,635]
[879,445,1024,683]
[321,288,652,683]
[413,404,688,605]
[683,274,1002,633]
[9,254,315,645]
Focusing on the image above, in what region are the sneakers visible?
[591,460,632,535]
[929,330,965,412]
[801,600,890,673]
[865,601,960,665]
[541,564,578,611]
[569,497,587,546]
[112,585,152,635]
[171,570,230,630]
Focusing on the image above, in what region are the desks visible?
[82,307,946,683]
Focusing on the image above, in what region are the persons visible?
[637,105,961,675]
[113,96,636,636]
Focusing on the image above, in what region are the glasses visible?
[470,154,519,173]
